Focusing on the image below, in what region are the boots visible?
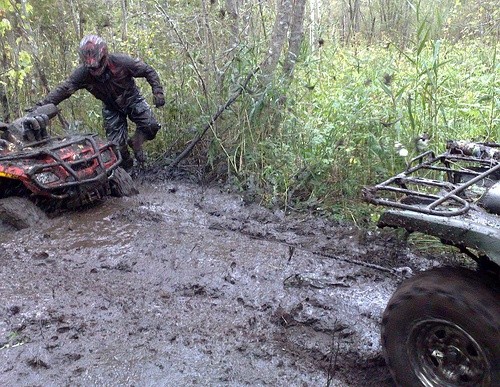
[126,166,133,177]
[128,129,147,162]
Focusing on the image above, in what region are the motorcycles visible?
[361,137,500,387]
[0,104,138,229]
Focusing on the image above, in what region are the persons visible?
[23,36,166,178]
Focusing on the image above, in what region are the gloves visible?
[23,105,38,112]
[152,93,164,108]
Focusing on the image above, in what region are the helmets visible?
[78,35,110,76]
